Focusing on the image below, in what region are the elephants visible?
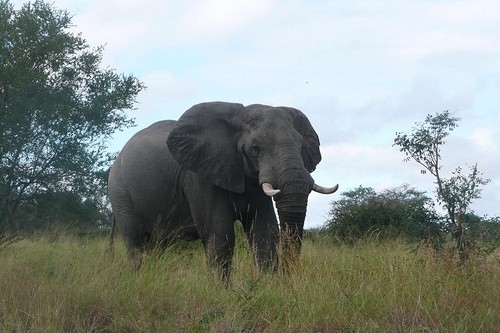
[107,101,338,289]
